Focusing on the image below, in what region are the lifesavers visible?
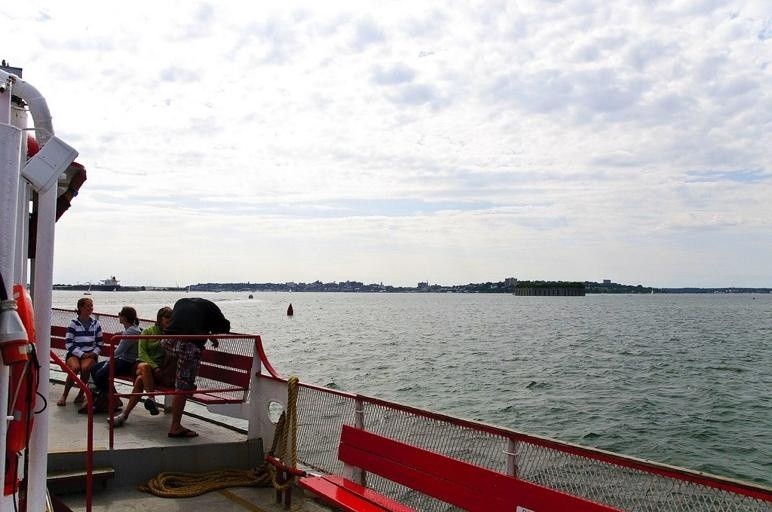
[8,282,39,453]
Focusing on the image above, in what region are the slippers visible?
[57,400,66,407]
[167,427,200,438]
[74,394,86,403]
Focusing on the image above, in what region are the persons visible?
[106,305,177,427]
[160,296,231,438]
[56,296,105,407]
[91,306,143,415]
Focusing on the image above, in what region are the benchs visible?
[49,324,254,411]
[293,422,617,512]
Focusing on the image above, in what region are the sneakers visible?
[106,413,127,426]
[144,399,160,416]
